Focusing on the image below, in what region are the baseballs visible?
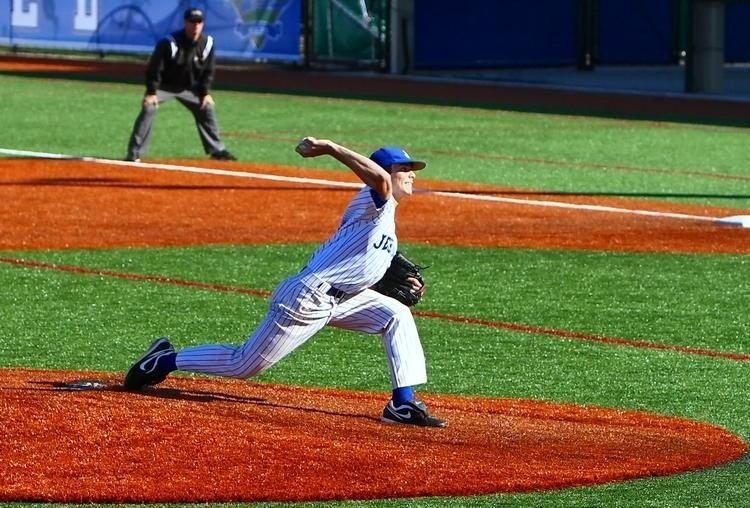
[299,136,317,150]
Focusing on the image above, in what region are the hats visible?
[369,147,426,174]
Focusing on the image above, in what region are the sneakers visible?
[124,337,175,390]
[122,157,142,163]
[380,399,449,429]
[209,150,237,161]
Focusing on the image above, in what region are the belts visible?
[299,265,346,298]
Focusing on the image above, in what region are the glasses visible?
[187,15,203,22]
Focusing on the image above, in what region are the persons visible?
[122,135,451,431]
[123,7,239,163]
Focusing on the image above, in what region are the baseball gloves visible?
[373,251,432,307]
